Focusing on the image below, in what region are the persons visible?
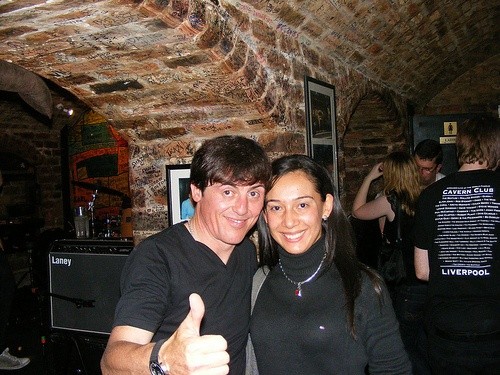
[99,134,274,375]
[247,153,414,374]
[0,175,31,371]
[351,112,500,374]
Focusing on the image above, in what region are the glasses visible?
[416,165,437,172]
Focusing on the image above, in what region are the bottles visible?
[88,213,99,238]
[74,207,89,239]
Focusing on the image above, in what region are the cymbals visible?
[72,178,125,197]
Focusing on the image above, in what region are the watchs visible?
[149,338,170,375]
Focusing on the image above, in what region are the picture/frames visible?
[165,164,197,227]
[303,74,341,203]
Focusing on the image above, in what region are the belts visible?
[432,325,500,343]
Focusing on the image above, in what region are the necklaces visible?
[278,222,331,298]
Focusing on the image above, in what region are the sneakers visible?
[1,347,31,370]
